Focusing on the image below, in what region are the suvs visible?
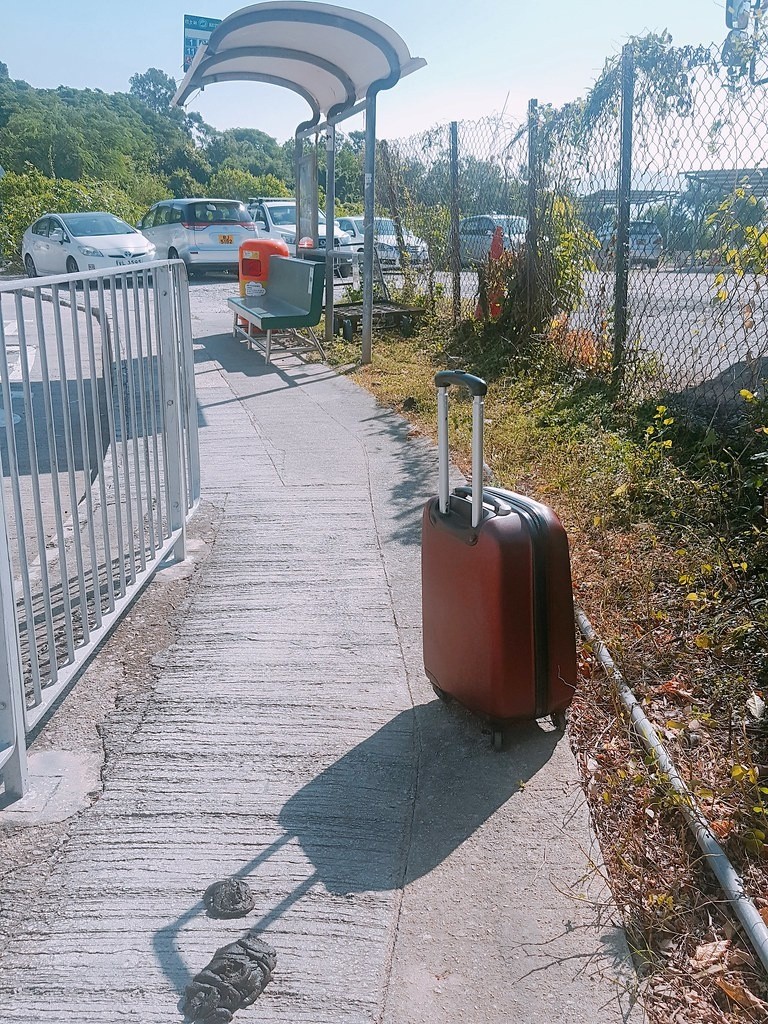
[241,197,353,278]
[447,215,551,269]
[135,197,261,278]
[589,219,663,272]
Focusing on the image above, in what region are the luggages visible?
[421,368,576,745]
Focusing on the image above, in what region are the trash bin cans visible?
[238,237,290,336]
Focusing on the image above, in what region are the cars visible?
[334,215,432,272]
[19,210,159,291]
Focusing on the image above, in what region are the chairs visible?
[211,210,224,221]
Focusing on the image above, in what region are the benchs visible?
[226,255,326,364]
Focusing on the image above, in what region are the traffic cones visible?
[475,225,507,321]
